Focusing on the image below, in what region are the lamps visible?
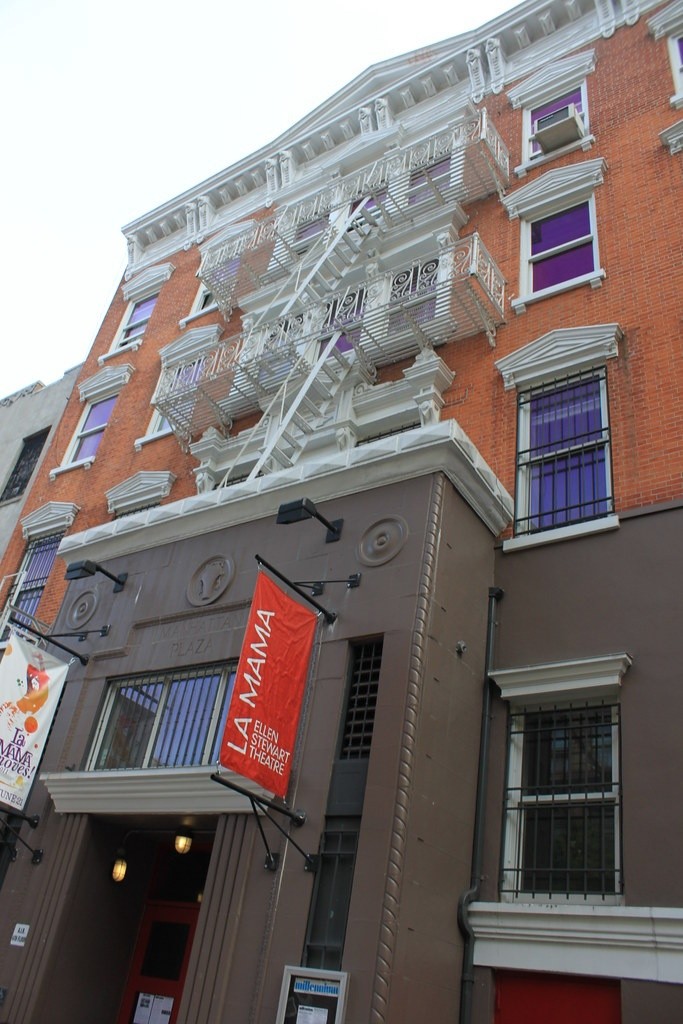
[276,497,344,543]
[175,824,195,854]
[64,560,128,594]
[112,847,128,883]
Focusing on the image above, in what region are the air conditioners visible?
[534,103,584,155]
